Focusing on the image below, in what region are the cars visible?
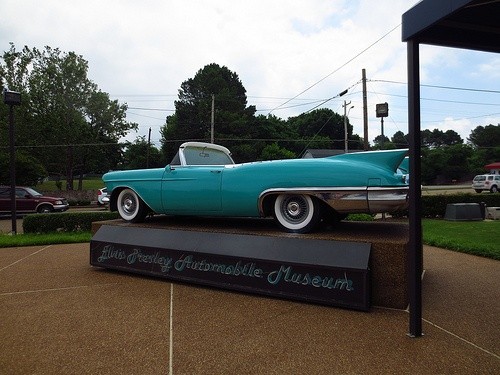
[98,142,410,233]
[0,187,70,217]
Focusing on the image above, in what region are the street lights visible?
[376,102,389,150]
[4,92,22,236]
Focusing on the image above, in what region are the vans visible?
[472,175,500,193]
